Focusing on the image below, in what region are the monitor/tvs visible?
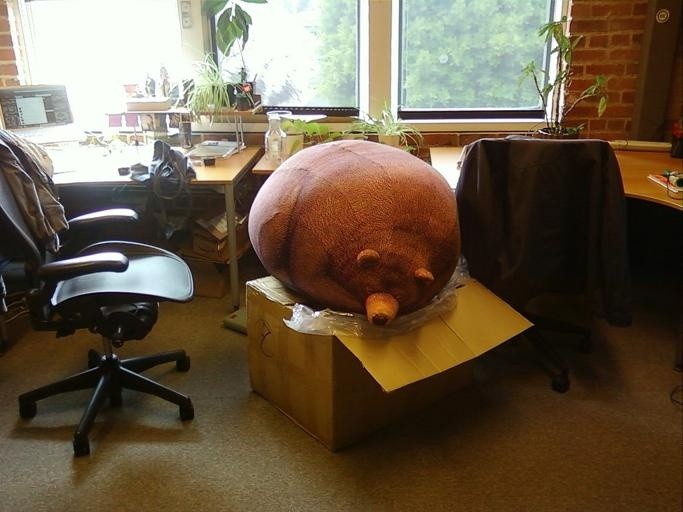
[0,83,78,144]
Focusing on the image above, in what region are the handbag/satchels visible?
[148,139,196,200]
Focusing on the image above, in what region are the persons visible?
[144,72,156,97]
[160,64,170,97]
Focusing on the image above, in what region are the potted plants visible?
[517,15,613,140]
[170,0,267,128]
[280,100,423,157]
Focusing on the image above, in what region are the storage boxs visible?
[244,268,535,453]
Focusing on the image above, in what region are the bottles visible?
[265,113,287,165]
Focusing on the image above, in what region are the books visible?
[126,97,171,110]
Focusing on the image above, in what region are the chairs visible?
[0,129,194,456]
[453,137,619,394]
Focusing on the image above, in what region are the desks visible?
[251,143,474,193]
[614,143,683,310]
[38,143,265,308]
